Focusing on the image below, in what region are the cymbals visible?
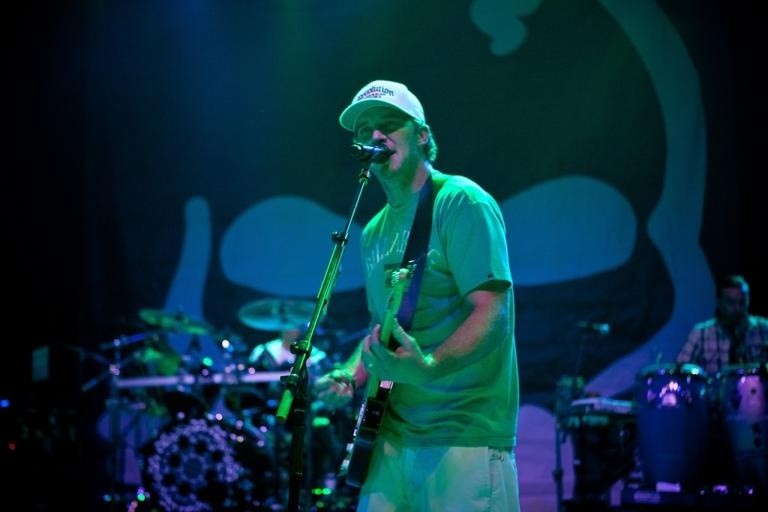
[239,299,315,332]
[138,309,210,335]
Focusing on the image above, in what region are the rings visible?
[364,364,374,369]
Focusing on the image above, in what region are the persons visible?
[306,79,521,512]
[674,273,768,377]
[257,328,327,367]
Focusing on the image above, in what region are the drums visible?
[145,417,269,511]
[156,358,217,411]
[716,364,767,426]
[222,365,269,411]
[642,363,710,418]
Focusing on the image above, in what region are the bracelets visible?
[342,371,355,390]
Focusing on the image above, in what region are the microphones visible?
[578,321,610,336]
[351,144,390,164]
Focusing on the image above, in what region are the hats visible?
[339,79,426,133]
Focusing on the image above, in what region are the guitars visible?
[338,261,416,499]
